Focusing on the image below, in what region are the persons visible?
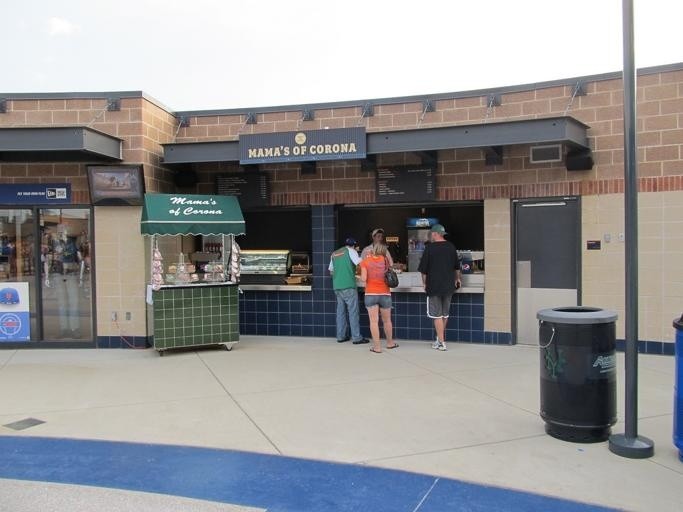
[361,229,393,267]
[359,240,399,353]
[44,223,85,339]
[328,237,370,344]
[417,223,462,351]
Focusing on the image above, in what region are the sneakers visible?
[337,336,369,344]
[432,339,447,351]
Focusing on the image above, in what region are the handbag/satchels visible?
[384,269,399,288]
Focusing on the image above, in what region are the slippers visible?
[369,343,400,353]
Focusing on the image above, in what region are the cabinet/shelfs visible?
[236,250,293,285]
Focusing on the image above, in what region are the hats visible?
[430,224,448,235]
[372,228,385,239]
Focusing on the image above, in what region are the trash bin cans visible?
[536,306,618,443]
[672,314,683,461]
